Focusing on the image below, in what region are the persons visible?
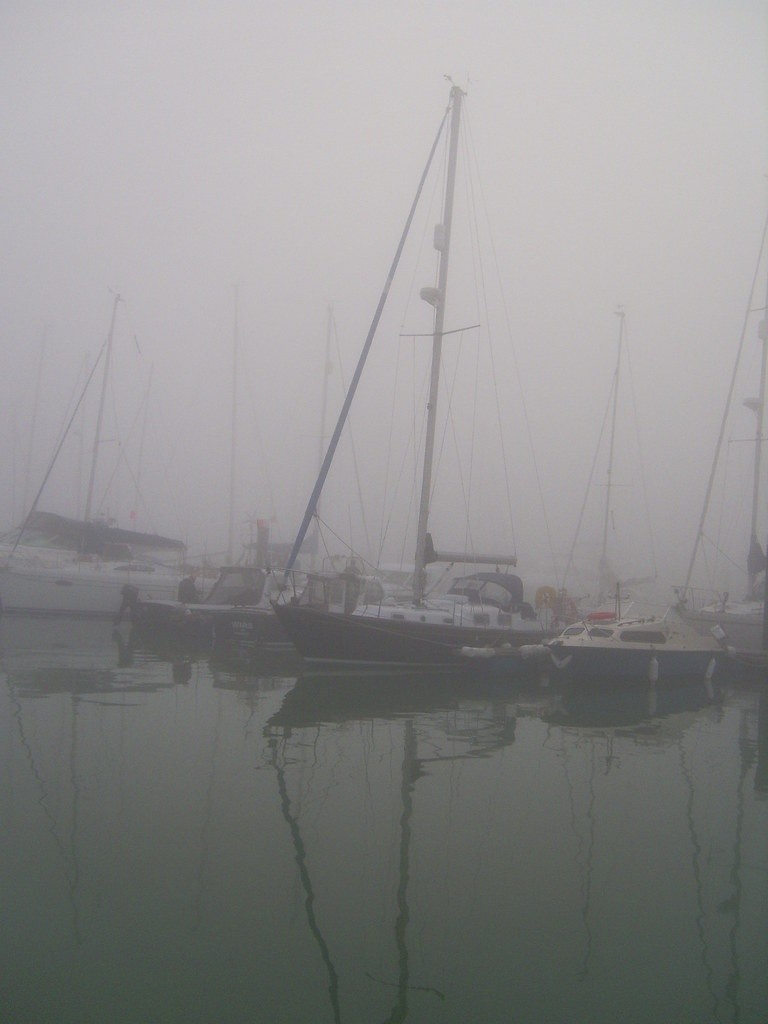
[178,571,198,607]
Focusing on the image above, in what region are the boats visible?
[112,511,297,646]
[544,584,720,710]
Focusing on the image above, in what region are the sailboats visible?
[268,64,544,662]
[542,305,703,625]
[680,215,768,668]
[0,288,218,613]
